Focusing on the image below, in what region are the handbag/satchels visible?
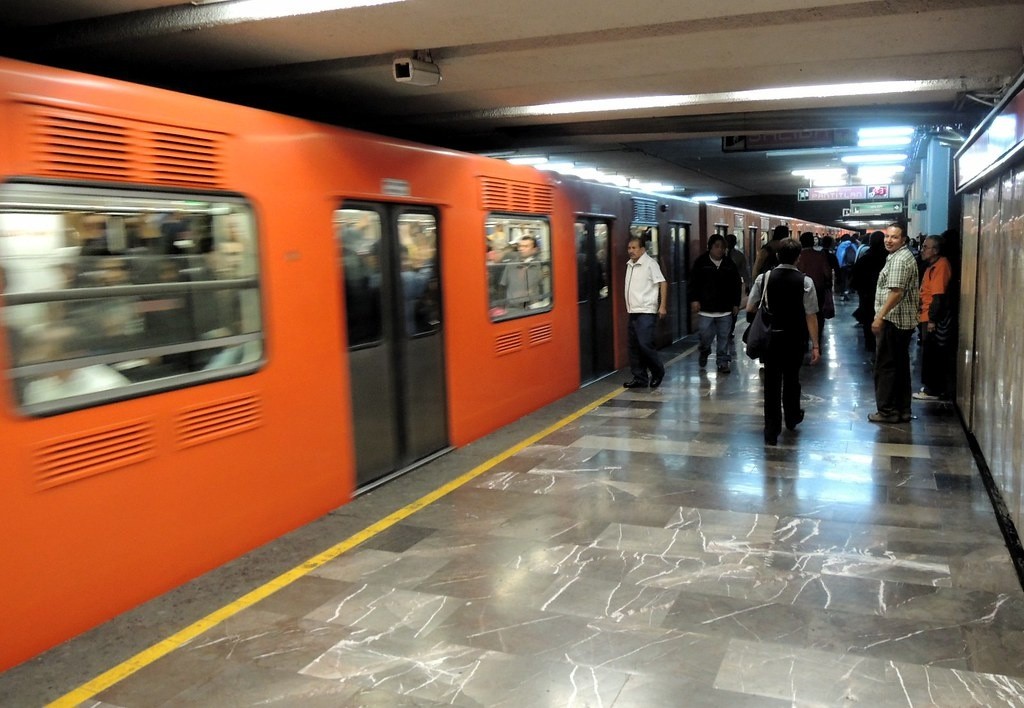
[746,307,778,360]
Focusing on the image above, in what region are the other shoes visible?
[699,353,708,367]
[903,413,912,423]
[868,412,901,423]
[766,438,778,445]
[844,294,850,301]
[790,409,804,430]
[717,364,731,374]
[913,392,939,399]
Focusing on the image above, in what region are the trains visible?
[2,56,877,671]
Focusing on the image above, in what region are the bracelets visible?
[812,347,819,349]
[875,312,885,320]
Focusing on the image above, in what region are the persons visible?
[623,236,668,389]
[687,234,743,373]
[752,225,790,283]
[867,222,920,423]
[797,231,835,355]
[811,233,928,353]
[0,214,542,403]
[911,235,953,400]
[744,239,820,445]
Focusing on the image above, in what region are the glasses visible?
[921,245,934,250]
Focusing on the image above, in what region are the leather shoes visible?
[623,378,648,388]
[650,371,665,387]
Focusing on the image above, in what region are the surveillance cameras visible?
[390,57,439,87]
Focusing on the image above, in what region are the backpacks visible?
[845,242,856,264]
